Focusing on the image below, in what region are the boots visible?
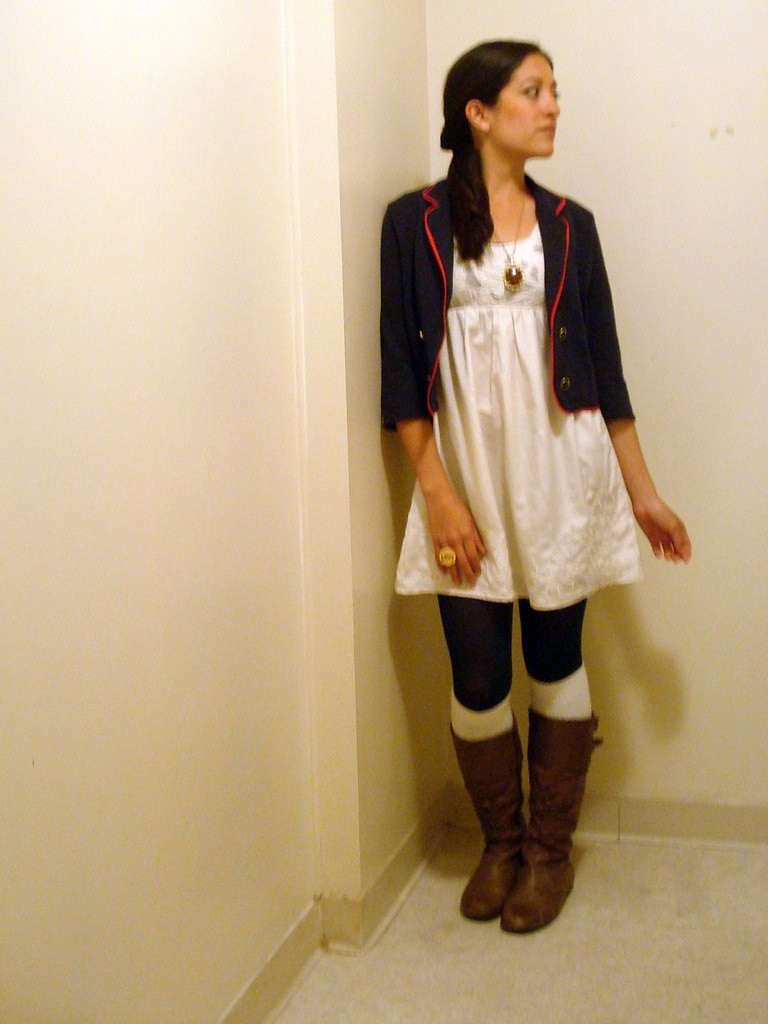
[500,706,598,934]
[450,708,529,921]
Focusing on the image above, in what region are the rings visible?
[439,547,456,567]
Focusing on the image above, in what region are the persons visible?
[380,40,692,932]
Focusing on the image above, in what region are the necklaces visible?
[494,191,525,291]
